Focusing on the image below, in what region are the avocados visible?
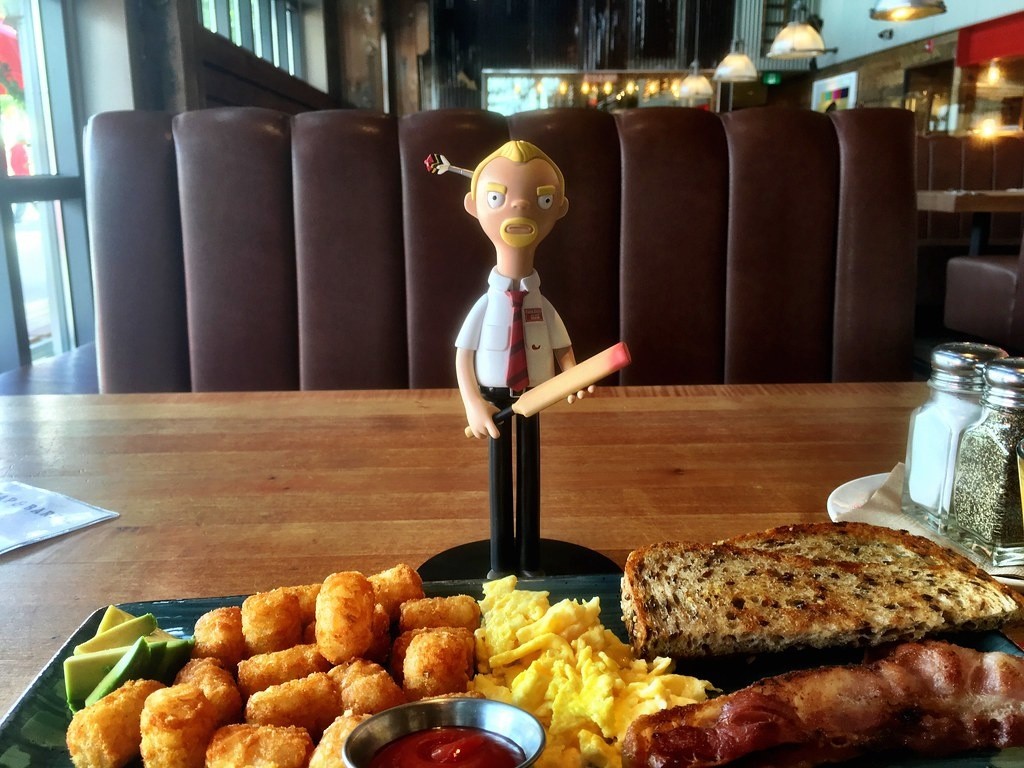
[62,604,195,715]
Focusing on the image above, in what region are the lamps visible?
[714,37,775,84]
[672,78,682,98]
[768,23,836,60]
[869,0,948,21]
[677,1,713,98]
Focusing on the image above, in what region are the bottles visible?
[936,355,1024,577]
[900,342,1011,537]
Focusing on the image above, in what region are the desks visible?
[0,368,1024,768]
[917,188,1024,255]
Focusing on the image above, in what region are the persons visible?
[454,141,594,580]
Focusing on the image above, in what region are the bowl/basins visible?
[343,697,545,767]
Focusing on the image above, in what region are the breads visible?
[619,521,1024,658]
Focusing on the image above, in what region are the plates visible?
[825,471,1024,587]
[0,573,1024,768]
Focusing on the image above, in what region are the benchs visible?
[77,104,1024,379]
